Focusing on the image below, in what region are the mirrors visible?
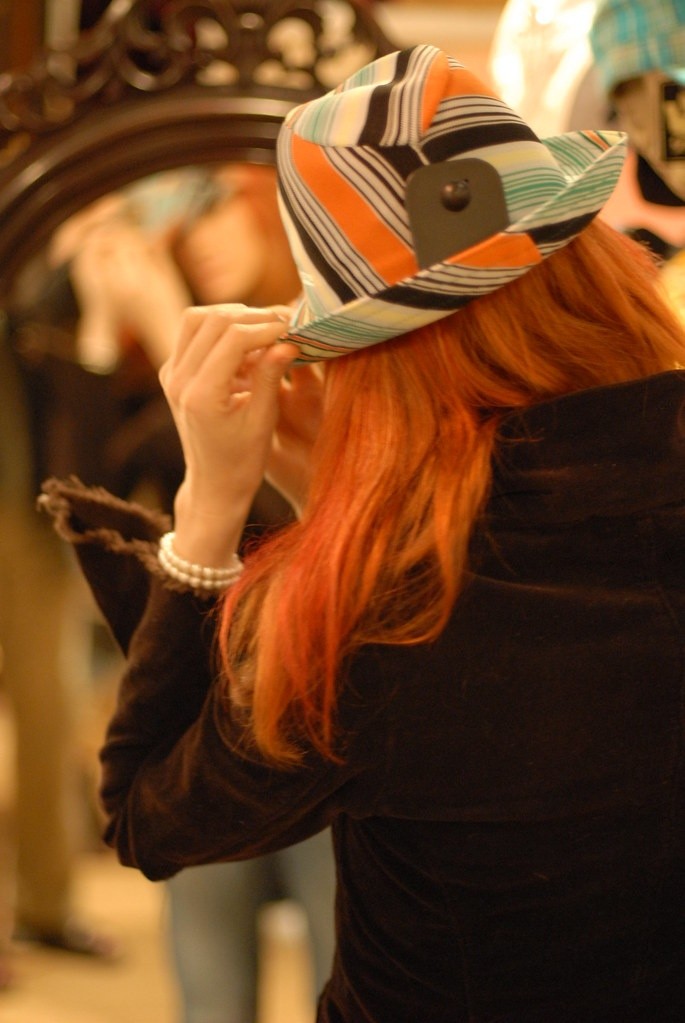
[0,0,411,1023]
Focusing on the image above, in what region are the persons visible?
[61,155,336,1023]
[40,45,685,1023]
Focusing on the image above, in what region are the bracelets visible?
[157,536,244,589]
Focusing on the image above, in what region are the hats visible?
[587,0,685,94]
[275,44,628,364]
